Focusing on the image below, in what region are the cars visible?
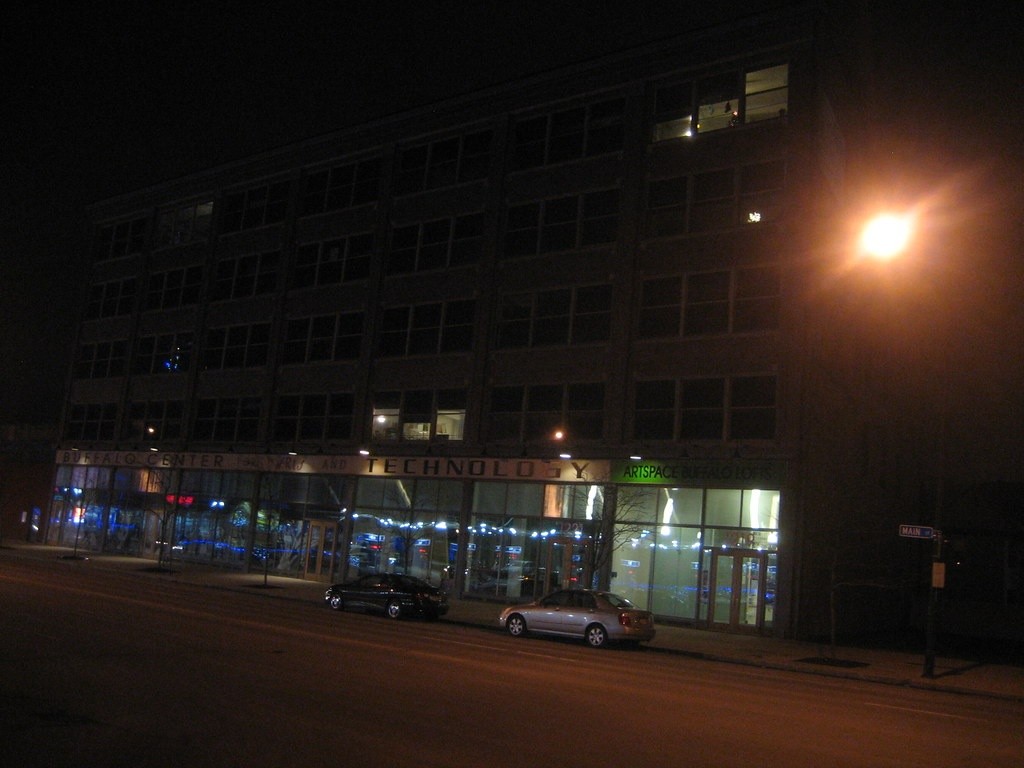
[498,588,658,649]
[324,572,451,621]
